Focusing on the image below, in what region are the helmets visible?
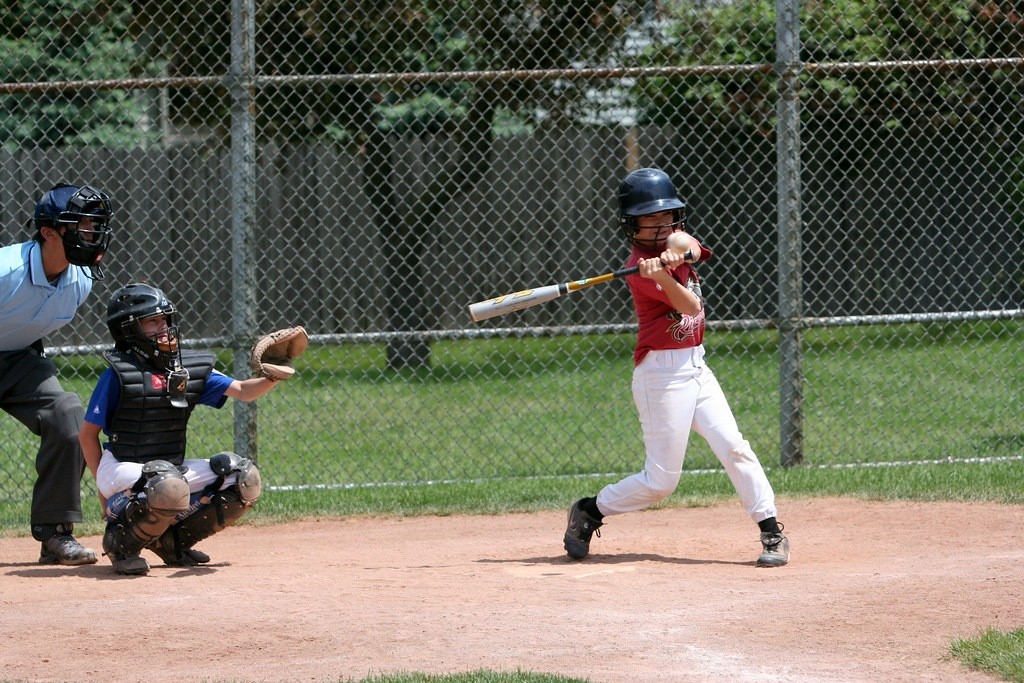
[618,168,687,253]
[108,283,184,370]
[35,182,114,267]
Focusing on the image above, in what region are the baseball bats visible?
[467,248,697,324]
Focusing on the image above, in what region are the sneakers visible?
[39,534,98,564]
[146,538,211,565]
[102,527,150,574]
[564,497,606,559]
[756,521,789,566]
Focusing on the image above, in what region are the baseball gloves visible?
[249,325,309,381]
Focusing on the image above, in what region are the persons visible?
[79,284,308,576]
[0,185,114,565]
[563,168,790,567]
